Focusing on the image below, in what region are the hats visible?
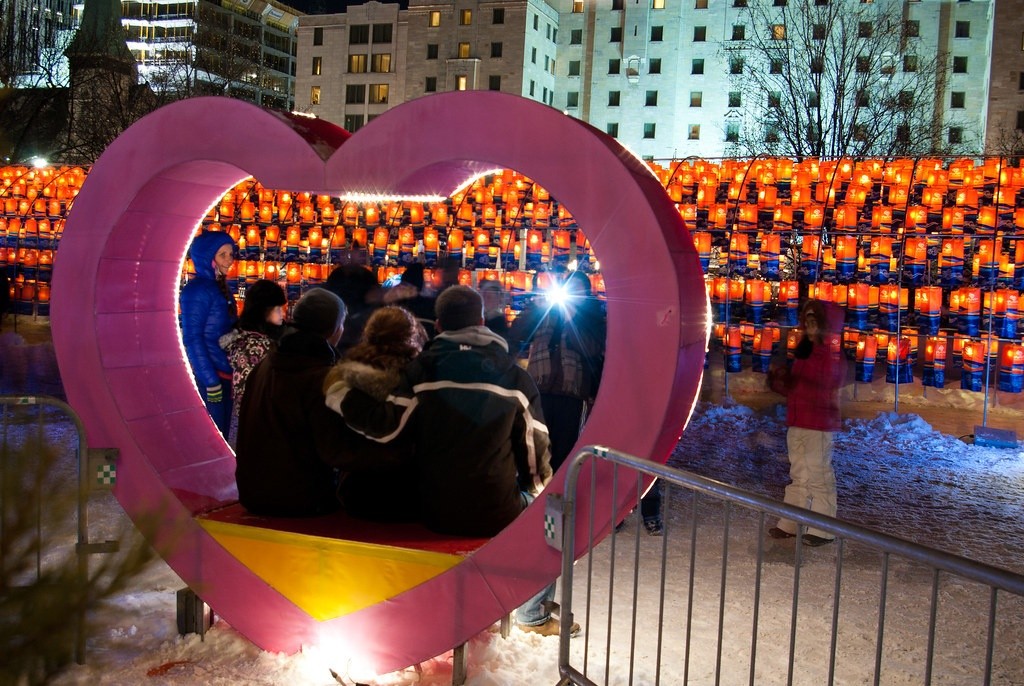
[245,280,286,305]
[401,262,425,292]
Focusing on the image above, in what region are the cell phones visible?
[772,342,780,358]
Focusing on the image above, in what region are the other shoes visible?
[643,519,665,536]
[517,617,581,638]
[614,519,625,532]
[767,527,796,538]
[801,534,834,547]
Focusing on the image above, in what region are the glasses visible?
[804,319,817,328]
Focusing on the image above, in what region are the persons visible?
[177,231,662,637]
[769,299,849,543]
